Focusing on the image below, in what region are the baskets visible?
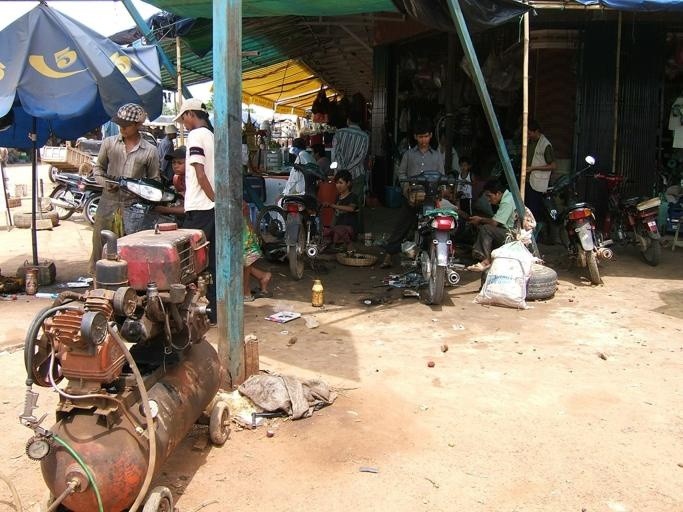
[335,253,378,266]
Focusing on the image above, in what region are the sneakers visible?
[467,262,490,273]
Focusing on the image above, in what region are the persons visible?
[275,116,370,254]
[397,119,558,270]
[87,94,271,329]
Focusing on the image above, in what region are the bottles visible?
[310,279,324,307]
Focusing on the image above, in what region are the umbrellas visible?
[1,2,164,286]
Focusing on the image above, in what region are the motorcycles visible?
[100,172,188,237]
[547,156,614,285]
[397,170,474,306]
[584,168,661,266]
[46,167,120,228]
[279,145,340,280]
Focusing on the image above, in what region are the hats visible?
[172,97,209,124]
[164,149,185,160]
[111,103,146,127]
[165,125,177,134]
